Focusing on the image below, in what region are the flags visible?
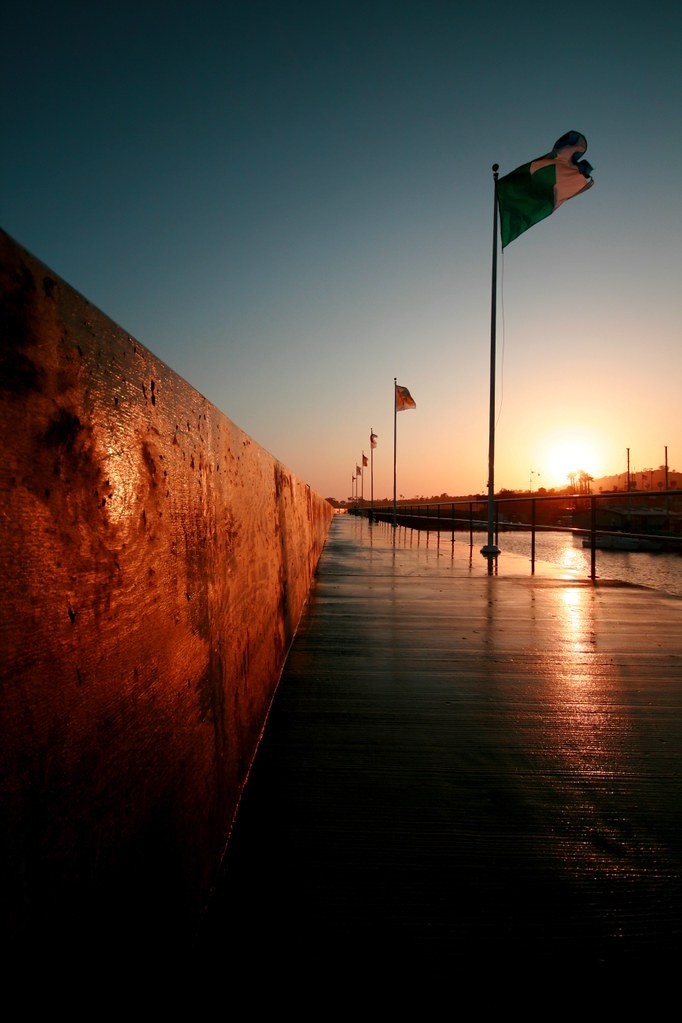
[363,455,368,466]
[497,130,595,247]
[370,434,378,448]
[357,467,361,475]
[396,385,416,412]
